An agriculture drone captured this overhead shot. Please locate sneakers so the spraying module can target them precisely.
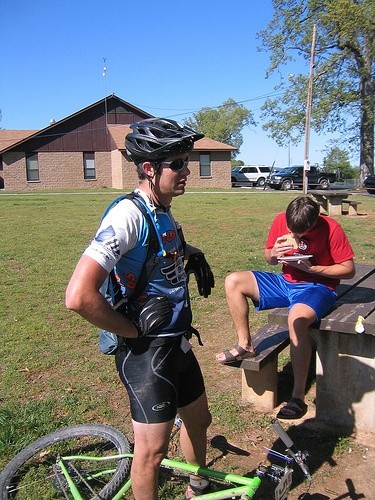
[185,481,238,500]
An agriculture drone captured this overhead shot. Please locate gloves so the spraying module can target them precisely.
[128,294,176,338]
[184,253,215,298]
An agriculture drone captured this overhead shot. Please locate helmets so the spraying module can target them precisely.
[123,117,205,162]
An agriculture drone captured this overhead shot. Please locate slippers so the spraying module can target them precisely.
[276,398,308,420]
[216,346,256,363]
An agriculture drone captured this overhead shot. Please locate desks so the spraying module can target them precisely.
[307,190,348,215]
[343,200,362,216]
[267,263,375,433]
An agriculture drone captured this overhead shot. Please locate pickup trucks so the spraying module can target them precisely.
[267,166,336,190]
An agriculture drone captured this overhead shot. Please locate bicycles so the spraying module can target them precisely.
[0,418,312,500]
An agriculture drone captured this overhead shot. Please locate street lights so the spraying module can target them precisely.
[102,57,107,134]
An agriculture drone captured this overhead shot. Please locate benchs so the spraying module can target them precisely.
[224,323,289,409]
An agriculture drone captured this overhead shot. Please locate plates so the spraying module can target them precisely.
[277,255,314,261]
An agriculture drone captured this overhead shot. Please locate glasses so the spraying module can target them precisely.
[154,156,190,171]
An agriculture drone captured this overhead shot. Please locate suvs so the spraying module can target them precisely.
[231,165,274,187]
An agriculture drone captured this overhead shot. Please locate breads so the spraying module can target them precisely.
[281,234,301,249]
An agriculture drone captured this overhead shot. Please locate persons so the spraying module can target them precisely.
[66,117,235,500]
[216,197,356,419]
